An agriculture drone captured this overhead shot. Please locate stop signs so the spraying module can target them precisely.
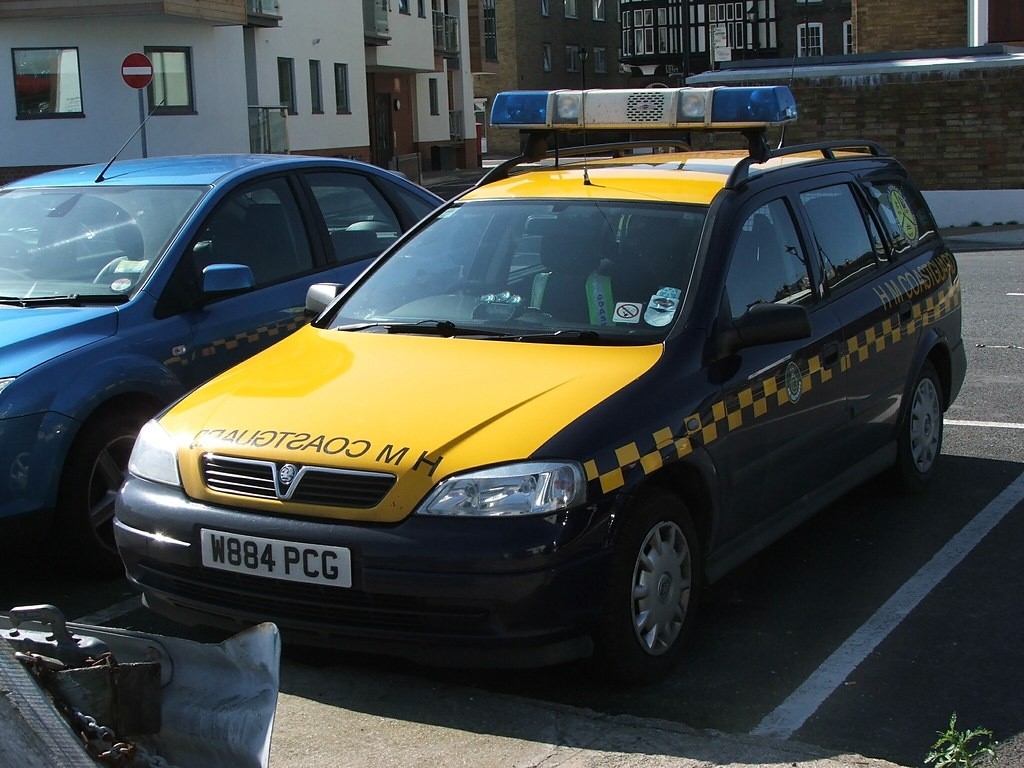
[122,52,153,90]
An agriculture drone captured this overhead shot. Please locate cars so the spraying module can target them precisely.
[116,90,966,684]
[0,152,449,563]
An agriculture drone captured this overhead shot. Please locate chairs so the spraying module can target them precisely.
[531,210,782,335]
